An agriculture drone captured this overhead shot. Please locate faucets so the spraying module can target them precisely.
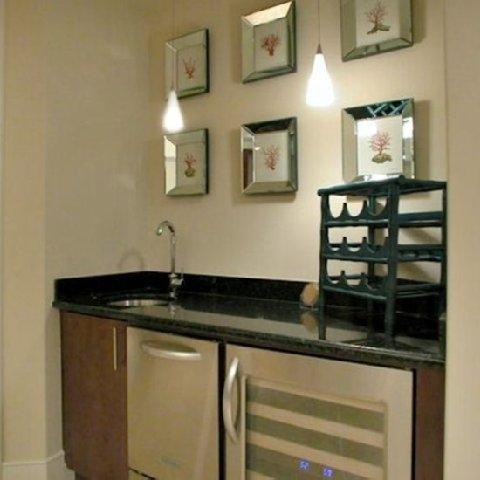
[155,221,185,292]
[166,302,179,322]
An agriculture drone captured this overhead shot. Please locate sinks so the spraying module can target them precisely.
[103,293,181,308]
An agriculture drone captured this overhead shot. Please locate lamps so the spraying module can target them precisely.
[301,0,337,109]
[162,1,186,134]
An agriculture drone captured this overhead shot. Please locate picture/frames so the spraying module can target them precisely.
[341,97,418,187]
[164,28,211,102]
[236,1,297,87]
[238,116,300,195]
[337,0,416,64]
[159,125,211,199]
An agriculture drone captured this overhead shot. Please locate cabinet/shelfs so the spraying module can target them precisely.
[57,308,128,480]
[313,178,449,348]
[219,339,415,480]
[130,323,228,479]
